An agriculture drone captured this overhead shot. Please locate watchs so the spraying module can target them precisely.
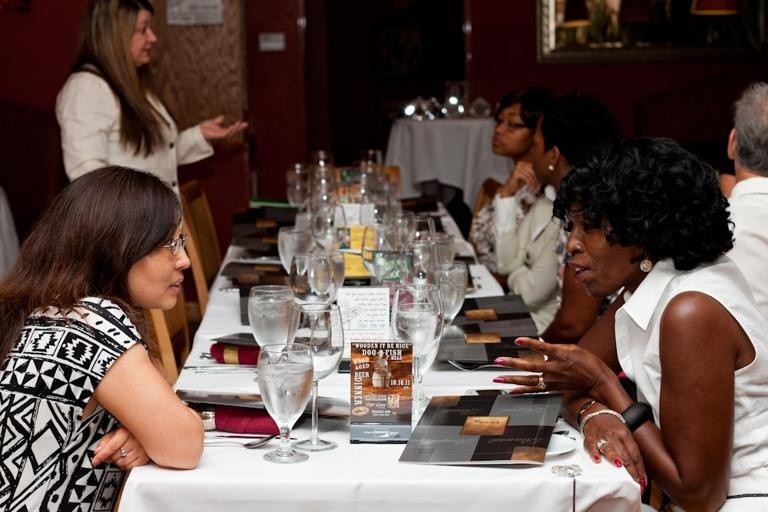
[577,399,596,426]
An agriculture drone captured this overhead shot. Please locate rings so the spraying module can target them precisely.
[535,376,548,390]
[596,438,609,450]
[120,447,127,457]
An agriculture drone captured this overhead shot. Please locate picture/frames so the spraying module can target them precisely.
[536,0,768,65]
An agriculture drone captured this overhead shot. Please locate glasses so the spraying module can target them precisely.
[494,116,526,133]
[158,233,189,256]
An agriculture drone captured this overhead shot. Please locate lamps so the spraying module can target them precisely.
[690,0,739,16]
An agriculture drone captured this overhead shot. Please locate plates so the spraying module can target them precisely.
[209,332,303,349]
[222,261,281,275]
[543,432,578,459]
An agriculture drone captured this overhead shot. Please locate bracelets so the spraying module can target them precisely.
[580,409,626,439]
[621,401,652,431]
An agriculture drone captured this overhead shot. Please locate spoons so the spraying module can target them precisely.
[201,434,278,450]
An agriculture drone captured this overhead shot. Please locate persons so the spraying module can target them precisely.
[467,83,558,266]
[1,164,202,512]
[530,92,618,343]
[724,86,768,305]
[492,137,768,511]
[492,158,557,335]
[55,0,248,207]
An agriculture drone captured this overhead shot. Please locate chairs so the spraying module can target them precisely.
[181,178,221,316]
[145,293,190,386]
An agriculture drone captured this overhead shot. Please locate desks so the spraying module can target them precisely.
[386,115,517,209]
[119,198,641,512]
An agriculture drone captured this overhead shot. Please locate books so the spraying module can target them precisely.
[400,388,566,469]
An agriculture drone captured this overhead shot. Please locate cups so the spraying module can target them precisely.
[391,283,443,421]
[383,212,415,254]
[436,260,467,327]
[256,342,314,465]
[359,226,397,288]
[358,196,390,225]
[288,302,344,453]
[432,234,455,269]
[313,251,346,297]
[290,254,335,357]
[278,226,312,277]
[310,204,346,254]
[405,217,434,245]
[247,285,295,368]
[394,302,437,408]
[403,239,433,285]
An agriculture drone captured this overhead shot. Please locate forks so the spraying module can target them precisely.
[447,358,516,372]
